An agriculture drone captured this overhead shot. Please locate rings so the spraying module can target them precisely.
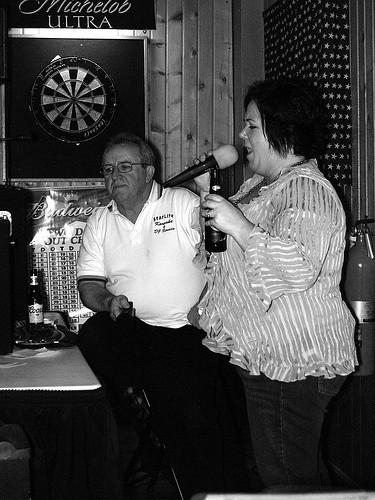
[205,211,212,218]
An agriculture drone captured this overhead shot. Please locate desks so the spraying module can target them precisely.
[0,312,107,500]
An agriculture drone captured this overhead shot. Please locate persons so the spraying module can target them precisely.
[183,77,361,497]
[77,134,250,500]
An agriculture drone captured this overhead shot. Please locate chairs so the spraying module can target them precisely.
[107,363,193,496]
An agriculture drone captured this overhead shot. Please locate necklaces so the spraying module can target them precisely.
[231,158,309,206]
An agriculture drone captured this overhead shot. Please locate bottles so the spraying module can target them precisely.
[26,269,44,343]
[205,169,226,252]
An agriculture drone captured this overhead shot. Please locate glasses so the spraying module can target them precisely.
[100,162,150,176]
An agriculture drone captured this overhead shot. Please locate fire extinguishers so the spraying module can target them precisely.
[342,216,375,377]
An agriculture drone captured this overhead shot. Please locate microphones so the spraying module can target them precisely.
[163,145,239,189]
[115,302,134,330]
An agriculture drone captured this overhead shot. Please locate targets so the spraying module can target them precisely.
[30,57,116,145]
